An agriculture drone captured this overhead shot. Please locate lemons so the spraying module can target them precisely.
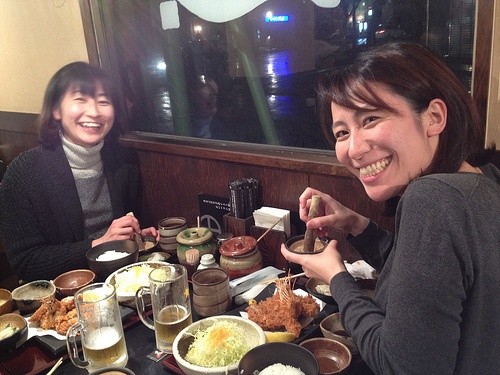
[264,331,296,343]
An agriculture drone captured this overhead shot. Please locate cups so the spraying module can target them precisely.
[191,269,233,317]
[66,282,128,374]
[135,264,193,355]
[158,216,188,261]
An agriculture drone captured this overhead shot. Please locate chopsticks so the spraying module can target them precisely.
[45,357,64,375]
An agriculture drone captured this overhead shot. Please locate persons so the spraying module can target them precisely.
[280,35,500,374]
[182,60,239,142]
[0,62,160,286]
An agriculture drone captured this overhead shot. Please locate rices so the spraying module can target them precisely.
[95,250,130,261]
[253,362,306,375]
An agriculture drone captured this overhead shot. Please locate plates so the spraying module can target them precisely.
[23,306,135,354]
[226,282,337,346]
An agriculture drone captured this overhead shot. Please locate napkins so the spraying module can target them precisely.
[344,259,378,280]
[252,206,291,238]
[229,266,287,307]
[13,295,77,349]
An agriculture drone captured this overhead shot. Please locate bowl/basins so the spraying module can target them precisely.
[54,269,95,298]
[319,312,360,355]
[173,315,267,375]
[12,280,57,314]
[86,240,138,281]
[0,314,27,349]
[238,341,319,375]
[105,261,169,309]
[0,288,13,316]
[88,367,135,375]
[306,277,336,306]
[138,252,170,265]
[299,338,352,375]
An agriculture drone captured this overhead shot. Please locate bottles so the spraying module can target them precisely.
[176,227,262,290]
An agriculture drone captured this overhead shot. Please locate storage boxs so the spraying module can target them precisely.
[222,212,255,238]
[357,278,377,291]
[251,224,304,271]
